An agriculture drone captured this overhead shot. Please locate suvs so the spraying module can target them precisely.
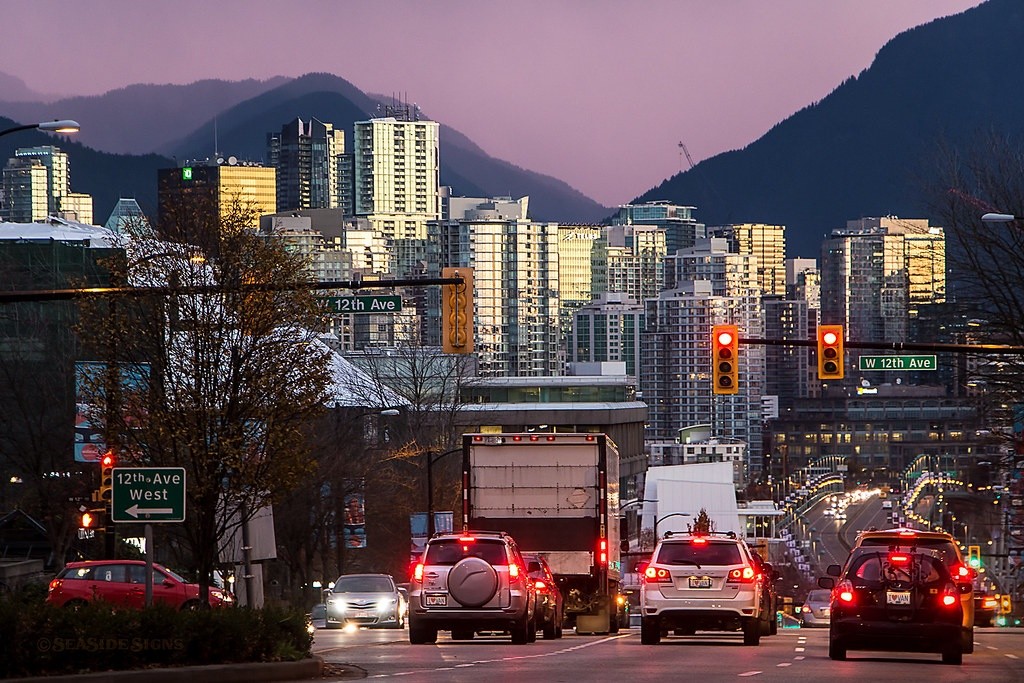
[639,531,768,646]
[409,530,541,646]
[826,526,979,654]
[43,560,236,613]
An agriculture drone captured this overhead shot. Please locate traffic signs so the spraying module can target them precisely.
[110,467,186,523]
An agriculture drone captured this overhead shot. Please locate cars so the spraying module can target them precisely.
[818,545,972,664]
[823,488,901,520]
[323,574,407,629]
[798,588,832,629]
[674,549,782,637]
[973,590,994,628]
[523,555,568,639]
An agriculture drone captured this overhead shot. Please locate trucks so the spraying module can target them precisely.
[460,432,630,636]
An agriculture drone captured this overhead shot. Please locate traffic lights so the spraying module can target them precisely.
[818,325,844,380]
[102,455,114,501]
[713,324,739,395]
[968,546,981,569]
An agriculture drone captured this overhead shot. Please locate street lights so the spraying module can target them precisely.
[653,512,691,551]
[334,400,401,577]
[770,453,847,567]
[898,453,992,551]
